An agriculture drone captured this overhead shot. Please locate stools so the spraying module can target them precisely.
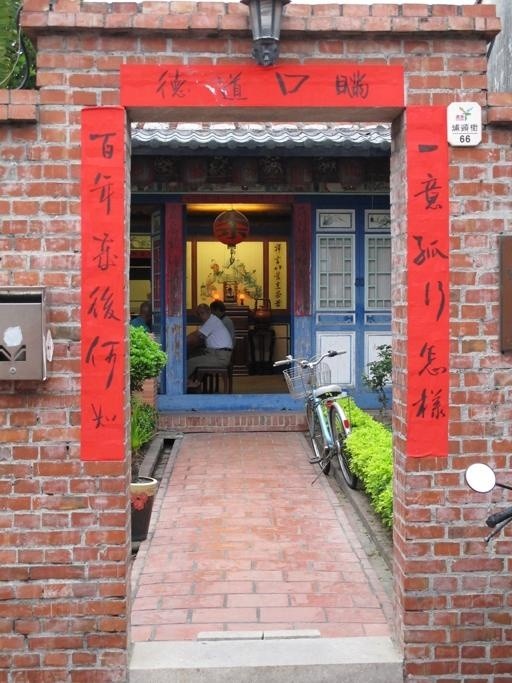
[197,367,233,393]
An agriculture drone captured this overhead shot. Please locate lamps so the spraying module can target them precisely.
[240,1,293,68]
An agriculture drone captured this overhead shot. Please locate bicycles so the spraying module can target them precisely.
[273,348,358,490]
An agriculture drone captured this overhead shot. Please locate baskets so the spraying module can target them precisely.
[283,363,331,399]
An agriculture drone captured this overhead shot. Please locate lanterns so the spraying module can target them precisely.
[213,209,249,255]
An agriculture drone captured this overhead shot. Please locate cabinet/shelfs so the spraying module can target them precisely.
[221,304,249,376]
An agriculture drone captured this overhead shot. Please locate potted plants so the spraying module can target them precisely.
[128,404,160,552]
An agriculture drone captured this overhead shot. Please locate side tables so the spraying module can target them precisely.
[246,328,275,375]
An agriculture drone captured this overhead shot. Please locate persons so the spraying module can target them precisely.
[131,300,152,332]
[186,302,233,387]
[209,298,236,347]
